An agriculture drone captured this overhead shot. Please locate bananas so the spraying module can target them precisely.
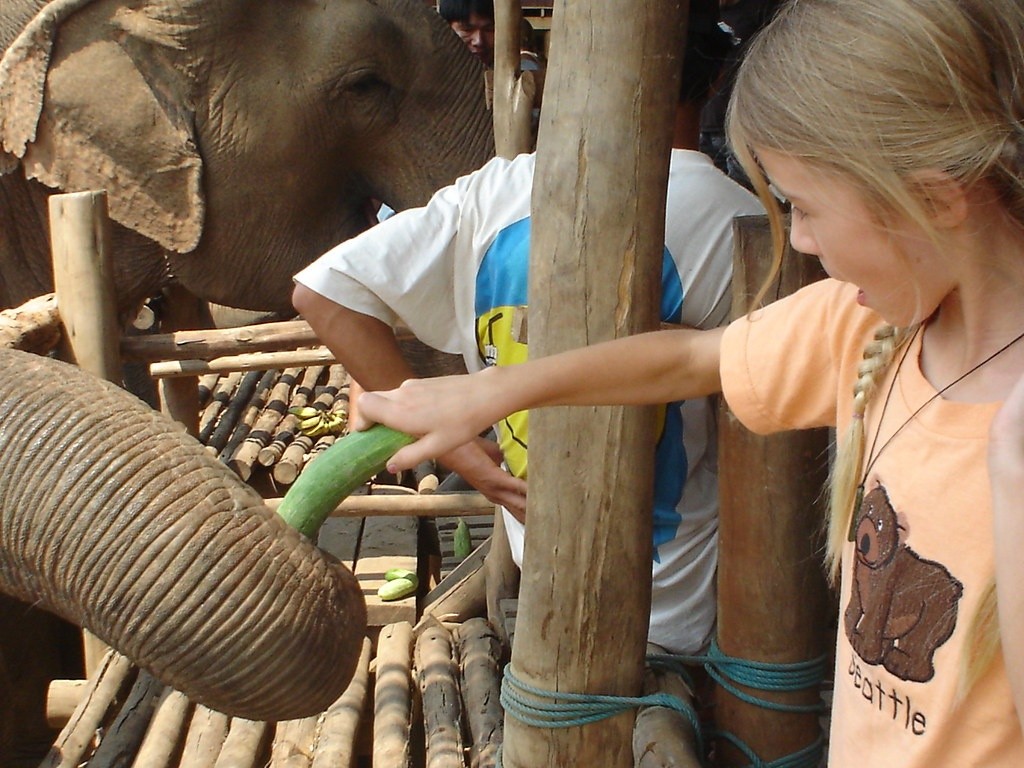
[286,406,348,435]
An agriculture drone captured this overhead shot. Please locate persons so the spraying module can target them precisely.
[348,0,1023,768]
[442,0,783,197]
[293,8,774,665]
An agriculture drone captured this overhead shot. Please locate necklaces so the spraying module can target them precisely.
[844,312,1022,540]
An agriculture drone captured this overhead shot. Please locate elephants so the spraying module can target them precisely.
[0,345,370,768]
[0,1,495,358]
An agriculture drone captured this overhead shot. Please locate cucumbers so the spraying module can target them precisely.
[277,424,419,541]
[454,520,471,558]
[378,569,419,600]
